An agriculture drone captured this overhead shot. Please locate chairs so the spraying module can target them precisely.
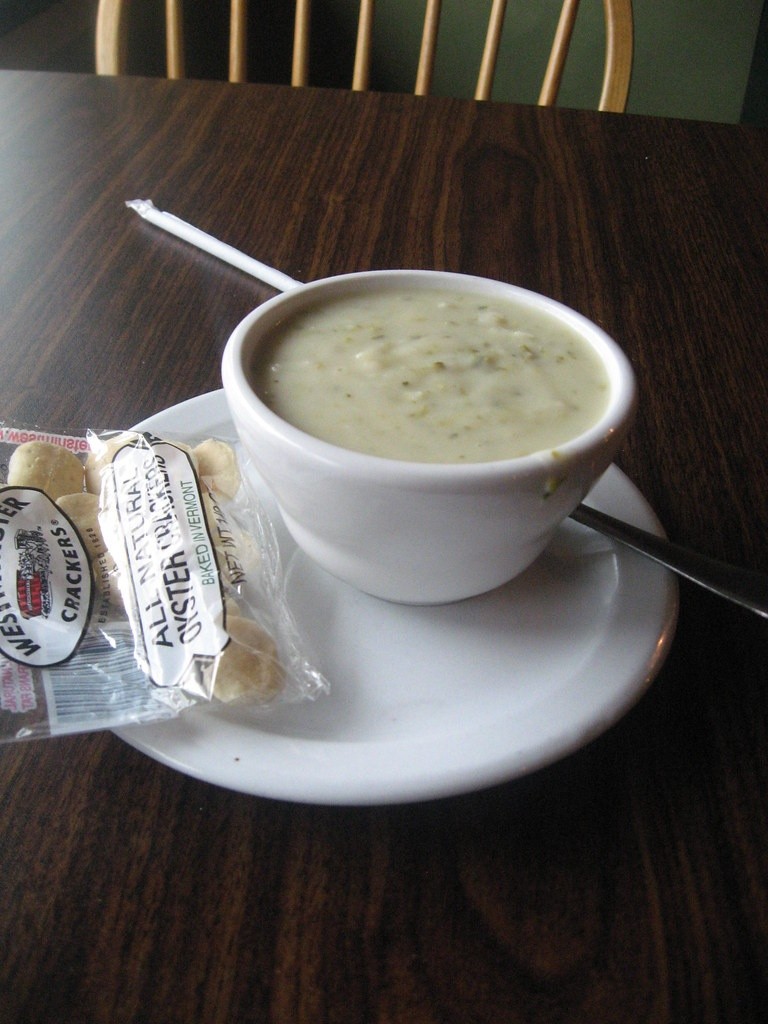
[94,1,636,113]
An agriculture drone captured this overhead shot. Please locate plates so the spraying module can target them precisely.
[109,384,684,805]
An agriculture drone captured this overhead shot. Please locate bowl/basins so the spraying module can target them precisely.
[219,270,640,606]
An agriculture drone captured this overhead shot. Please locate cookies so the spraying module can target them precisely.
[6,435,285,707]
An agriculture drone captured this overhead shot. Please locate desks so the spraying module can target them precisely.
[0,67,768,1024]
